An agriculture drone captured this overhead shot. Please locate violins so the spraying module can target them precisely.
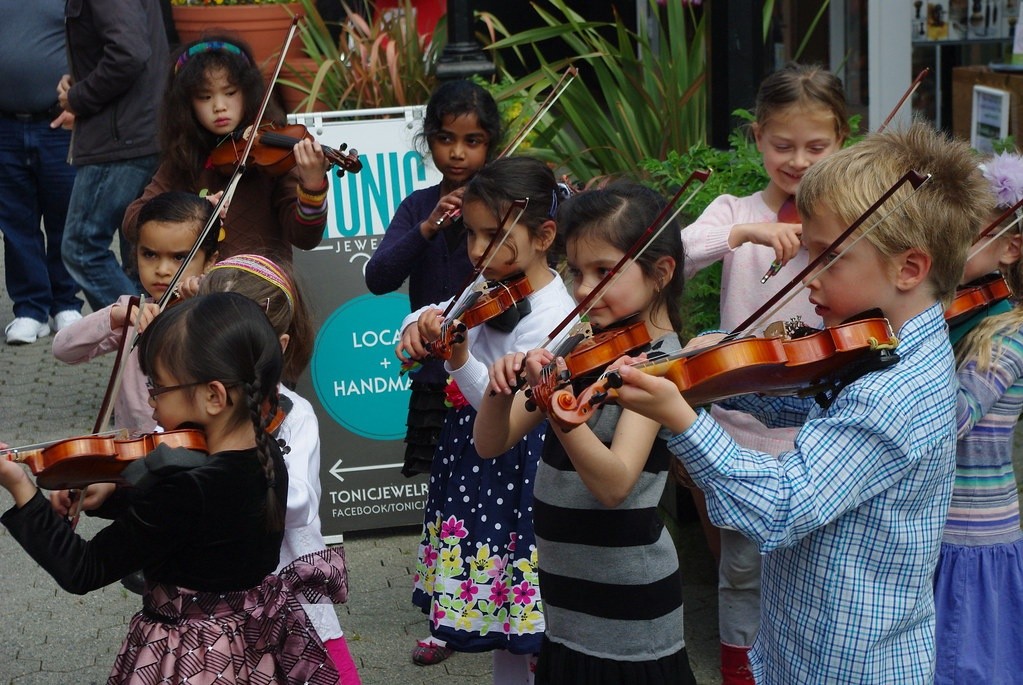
[944,269,1013,327]
[778,194,803,247]
[524,319,653,413]
[0,427,210,490]
[545,316,902,435]
[211,118,363,179]
[425,275,535,361]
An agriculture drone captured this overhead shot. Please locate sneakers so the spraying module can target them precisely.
[51,310,83,334]
[4,315,50,344]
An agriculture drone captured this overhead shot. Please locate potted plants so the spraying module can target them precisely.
[641,101,868,562]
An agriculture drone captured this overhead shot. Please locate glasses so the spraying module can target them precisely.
[144,381,234,407]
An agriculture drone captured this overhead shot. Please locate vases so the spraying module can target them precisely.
[172,2,333,112]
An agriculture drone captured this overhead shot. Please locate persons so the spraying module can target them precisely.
[51,0,177,310]
[677,64,848,685]
[473,178,697,685]
[364,79,501,665]
[605,118,992,685]
[51,191,223,438]
[0,294,349,685]
[121,31,331,264]
[931,154,1023,685]
[397,154,586,685]
[0,0,83,344]
[196,251,363,685]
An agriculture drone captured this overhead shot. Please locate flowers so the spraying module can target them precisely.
[170,0,297,6]
[978,147,1023,208]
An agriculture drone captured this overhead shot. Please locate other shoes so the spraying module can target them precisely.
[413,633,454,666]
